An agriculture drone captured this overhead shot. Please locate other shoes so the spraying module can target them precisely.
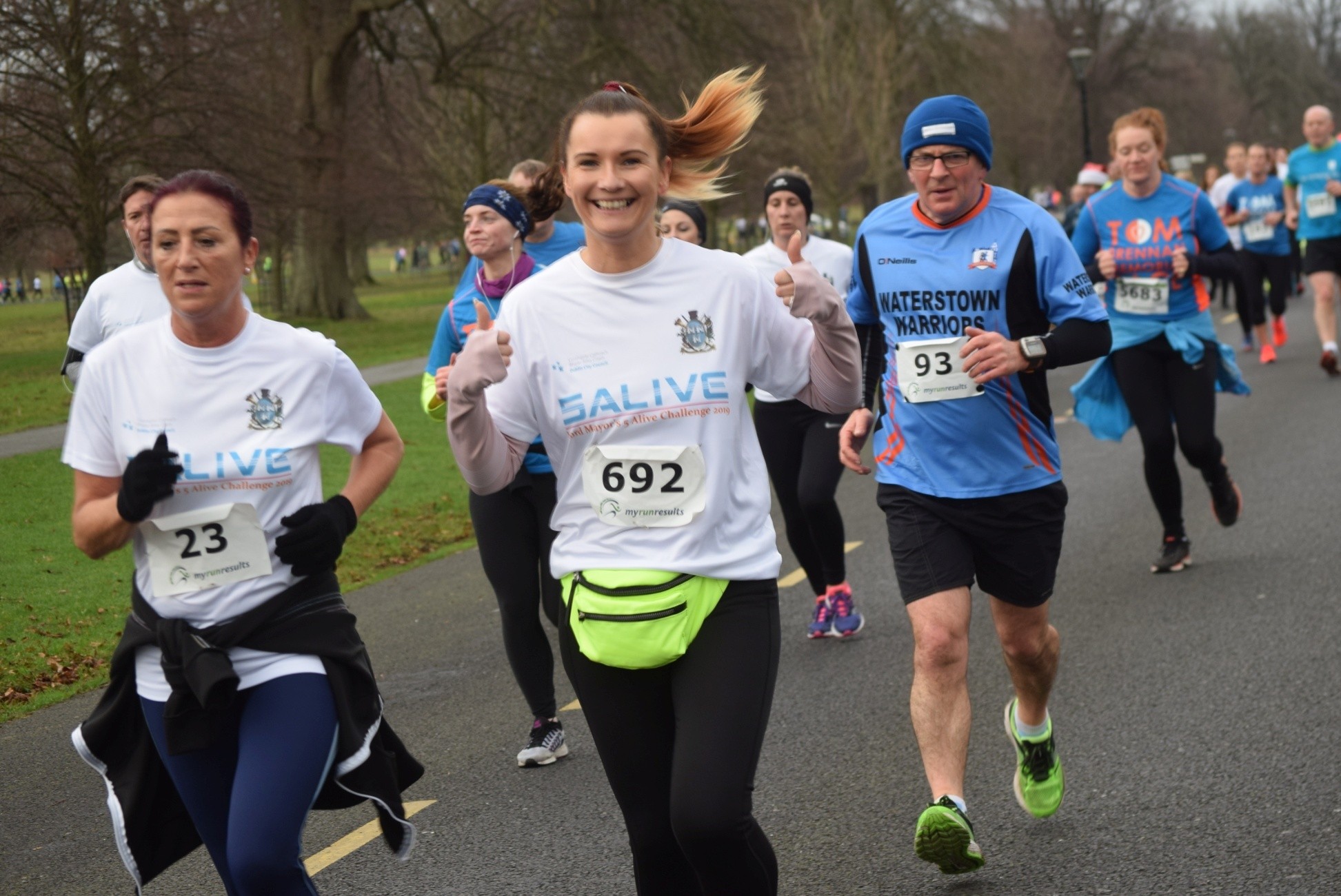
[1321,350,1341,375]
[1271,316,1285,347]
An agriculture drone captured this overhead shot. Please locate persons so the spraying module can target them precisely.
[0,277,14,302]
[55,273,65,296]
[61,172,406,896]
[32,276,43,299]
[735,166,866,641]
[1027,163,1112,238]
[1072,108,1242,575]
[1164,141,1308,365]
[1283,104,1341,378]
[60,176,253,380]
[394,237,460,269]
[419,167,570,767]
[453,159,585,297]
[839,95,1113,874]
[447,68,863,896]
[15,277,24,301]
[659,198,706,247]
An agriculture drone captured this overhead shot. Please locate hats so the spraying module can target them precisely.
[900,95,991,171]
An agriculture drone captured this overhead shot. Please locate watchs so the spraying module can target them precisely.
[1020,336,1047,372]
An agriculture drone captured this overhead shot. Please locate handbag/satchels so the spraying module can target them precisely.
[1259,344,1276,364]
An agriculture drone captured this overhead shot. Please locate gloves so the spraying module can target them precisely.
[116,433,183,524]
[272,493,361,577]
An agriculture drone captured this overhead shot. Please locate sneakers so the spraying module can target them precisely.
[913,797,985,874]
[1203,466,1242,527]
[515,714,570,766]
[1002,700,1064,818]
[1149,535,1190,574]
[806,595,833,638]
[824,583,863,638]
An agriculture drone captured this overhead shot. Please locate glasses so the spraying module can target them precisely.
[907,150,972,171]
